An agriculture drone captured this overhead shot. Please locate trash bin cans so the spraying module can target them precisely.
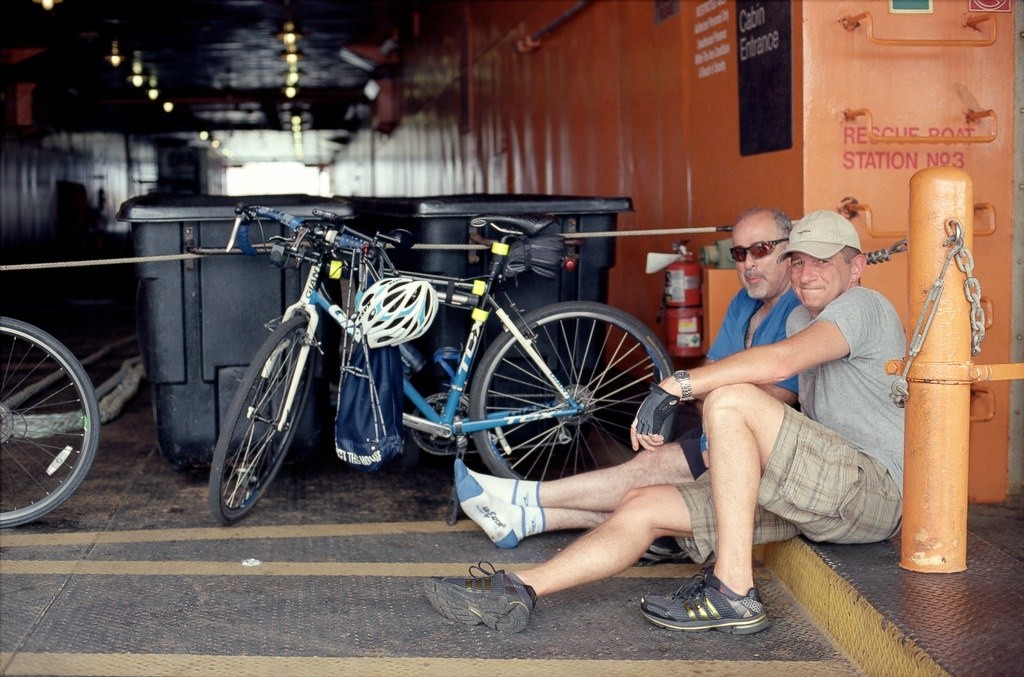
[351,195,636,483]
[115,192,359,472]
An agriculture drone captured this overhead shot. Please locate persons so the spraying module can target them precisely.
[454,208,813,563]
[423,209,909,635]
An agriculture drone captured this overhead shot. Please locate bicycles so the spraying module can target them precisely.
[208,200,680,525]
[0,317,102,528]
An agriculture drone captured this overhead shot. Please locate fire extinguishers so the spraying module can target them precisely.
[657,240,704,358]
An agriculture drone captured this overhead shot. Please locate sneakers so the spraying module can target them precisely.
[639,566,771,633]
[425,560,539,632]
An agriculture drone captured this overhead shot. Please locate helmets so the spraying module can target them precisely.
[357,278,439,348]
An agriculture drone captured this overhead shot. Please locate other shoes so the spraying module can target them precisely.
[642,534,694,565]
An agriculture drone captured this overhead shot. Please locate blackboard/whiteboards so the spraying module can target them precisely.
[736,0,793,157]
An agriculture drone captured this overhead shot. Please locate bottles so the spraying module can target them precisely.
[370,315,427,378]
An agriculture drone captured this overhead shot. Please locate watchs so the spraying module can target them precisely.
[673,369,692,401]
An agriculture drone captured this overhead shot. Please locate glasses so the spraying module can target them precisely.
[730,238,790,262]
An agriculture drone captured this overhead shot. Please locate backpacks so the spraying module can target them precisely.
[334,249,405,467]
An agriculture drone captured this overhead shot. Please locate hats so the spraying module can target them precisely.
[777,210,862,265]
